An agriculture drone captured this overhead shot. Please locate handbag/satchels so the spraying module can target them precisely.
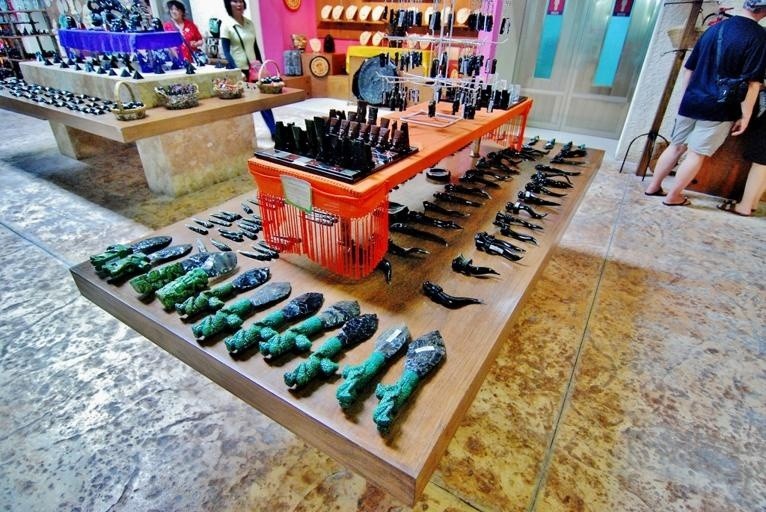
[191,49,208,64]
[717,77,747,102]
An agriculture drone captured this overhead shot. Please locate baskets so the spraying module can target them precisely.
[667,27,704,48]
[110,60,285,120]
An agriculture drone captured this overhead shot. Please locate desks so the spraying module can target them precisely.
[0,80,306,197]
[66,139,607,510]
[58,28,183,72]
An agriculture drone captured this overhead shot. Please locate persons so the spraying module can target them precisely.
[645,0,766,216]
[220,0,279,141]
[163,0,204,62]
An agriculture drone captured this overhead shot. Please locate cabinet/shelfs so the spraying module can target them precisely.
[0,9,62,78]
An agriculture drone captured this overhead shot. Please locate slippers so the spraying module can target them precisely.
[645,187,691,206]
[717,200,756,216]
